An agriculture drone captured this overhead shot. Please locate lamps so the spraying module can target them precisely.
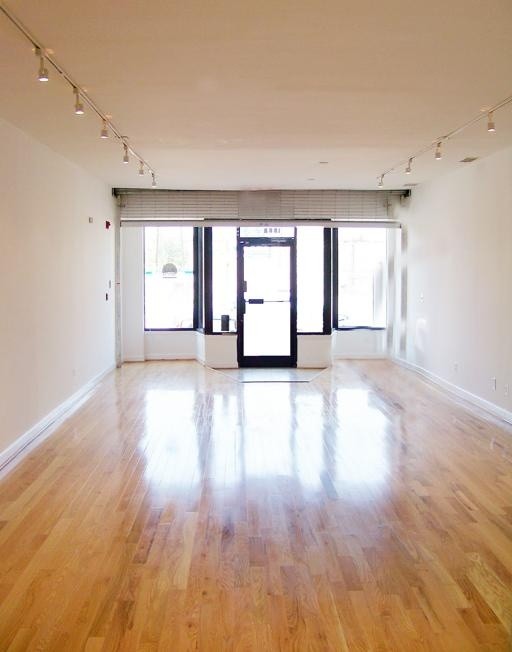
[0,3,161,188]
[372,97,512,188]
